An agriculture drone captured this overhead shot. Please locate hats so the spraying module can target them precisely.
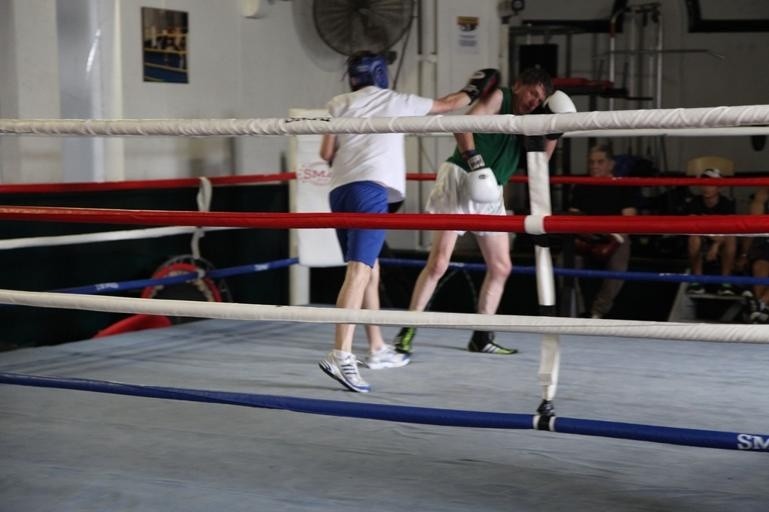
[701,167,722,178]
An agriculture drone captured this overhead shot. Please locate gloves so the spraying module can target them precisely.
[540,89,579,140]
[460,68,502,106]
[461,149,502,204]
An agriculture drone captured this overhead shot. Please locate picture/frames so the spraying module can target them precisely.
[684,1,769,34]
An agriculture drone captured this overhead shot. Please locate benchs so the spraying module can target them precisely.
[456,243,688,264]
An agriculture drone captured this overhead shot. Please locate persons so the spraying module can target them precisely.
[568,141,639,319]
[394,64,578,355]
[686,168,738,296]
[736,186,769,323]
[318,51,502,394]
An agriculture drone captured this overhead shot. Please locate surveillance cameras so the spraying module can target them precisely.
[498,0,526,18]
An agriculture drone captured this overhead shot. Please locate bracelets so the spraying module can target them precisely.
[741,253,747,257]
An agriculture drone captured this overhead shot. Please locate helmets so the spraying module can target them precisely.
[347,51,389,92]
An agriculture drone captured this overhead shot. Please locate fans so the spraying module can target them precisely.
[313,0,414,57]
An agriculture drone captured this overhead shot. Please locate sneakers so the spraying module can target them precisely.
[468,336,518,355]
[362,342,413,370]
[318,348,372,394]
[687,281,769,324]
[392,327,417,352]
